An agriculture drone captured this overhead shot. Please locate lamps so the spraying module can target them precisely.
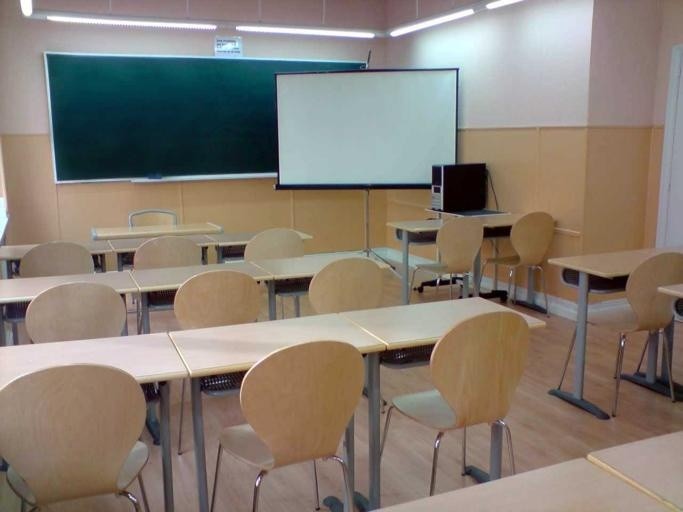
[16,1,520,40]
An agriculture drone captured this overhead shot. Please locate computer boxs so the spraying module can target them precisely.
[432,163,486,210]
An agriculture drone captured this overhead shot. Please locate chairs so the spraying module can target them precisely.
[2,212,681,512]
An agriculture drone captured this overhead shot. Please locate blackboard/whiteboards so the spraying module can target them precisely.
[43,51,369,184]
